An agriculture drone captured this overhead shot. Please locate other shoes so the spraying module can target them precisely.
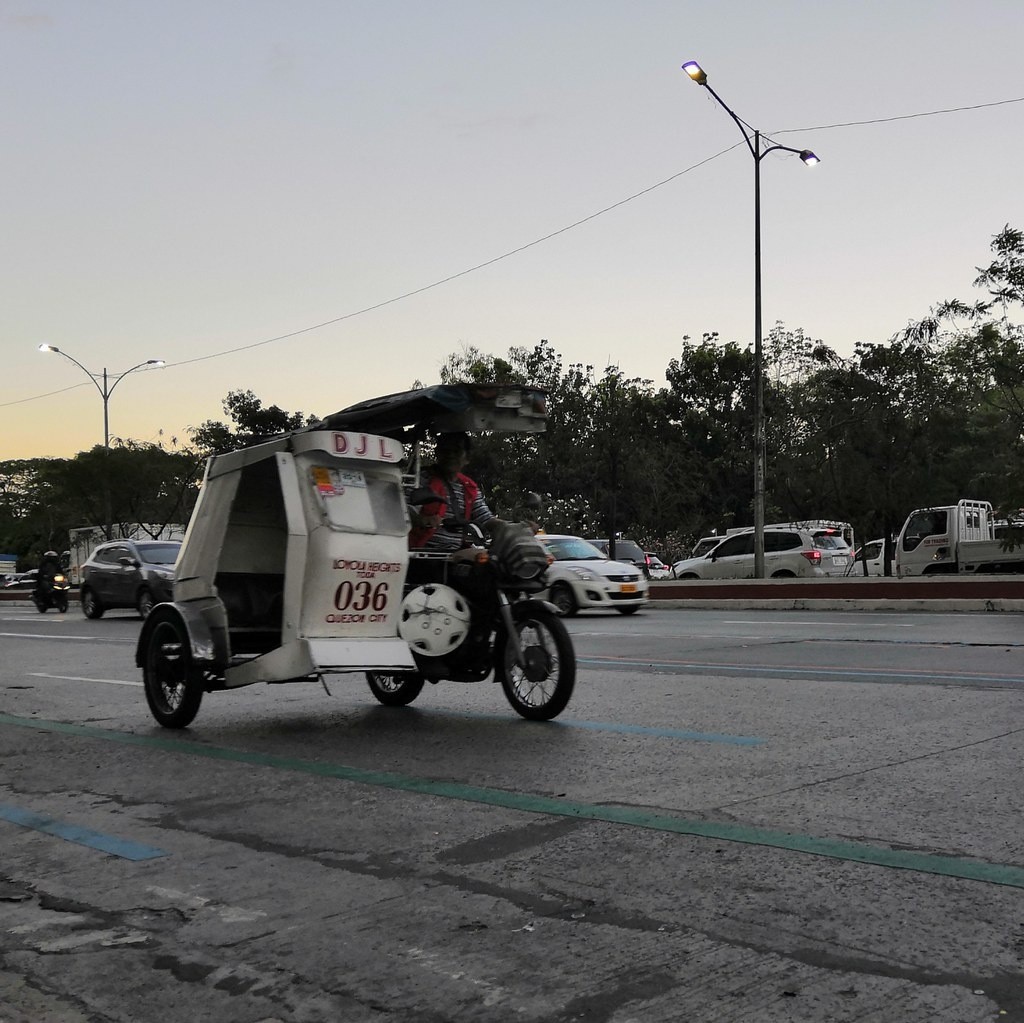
[413,653,450,677]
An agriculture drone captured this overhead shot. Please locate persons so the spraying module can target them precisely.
[406,432,513,677]
[35,551,65,606]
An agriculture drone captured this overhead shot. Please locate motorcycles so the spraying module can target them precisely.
[136,431,576,729]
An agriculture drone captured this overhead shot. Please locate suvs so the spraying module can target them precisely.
[668,526,824,580]
[79,538,186,619]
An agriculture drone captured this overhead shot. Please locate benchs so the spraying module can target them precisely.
[214,570,286,646]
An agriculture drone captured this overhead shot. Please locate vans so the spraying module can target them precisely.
[855,536,920,576]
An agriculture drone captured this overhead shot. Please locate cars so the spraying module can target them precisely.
[534,535,667,618]
[3,569,70,589]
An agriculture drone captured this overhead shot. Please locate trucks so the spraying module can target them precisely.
[895,499,1024,576]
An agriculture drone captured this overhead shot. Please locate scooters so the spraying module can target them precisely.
[26,569,72,613]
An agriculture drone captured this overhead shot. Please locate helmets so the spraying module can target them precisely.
[44,551,58,557]
